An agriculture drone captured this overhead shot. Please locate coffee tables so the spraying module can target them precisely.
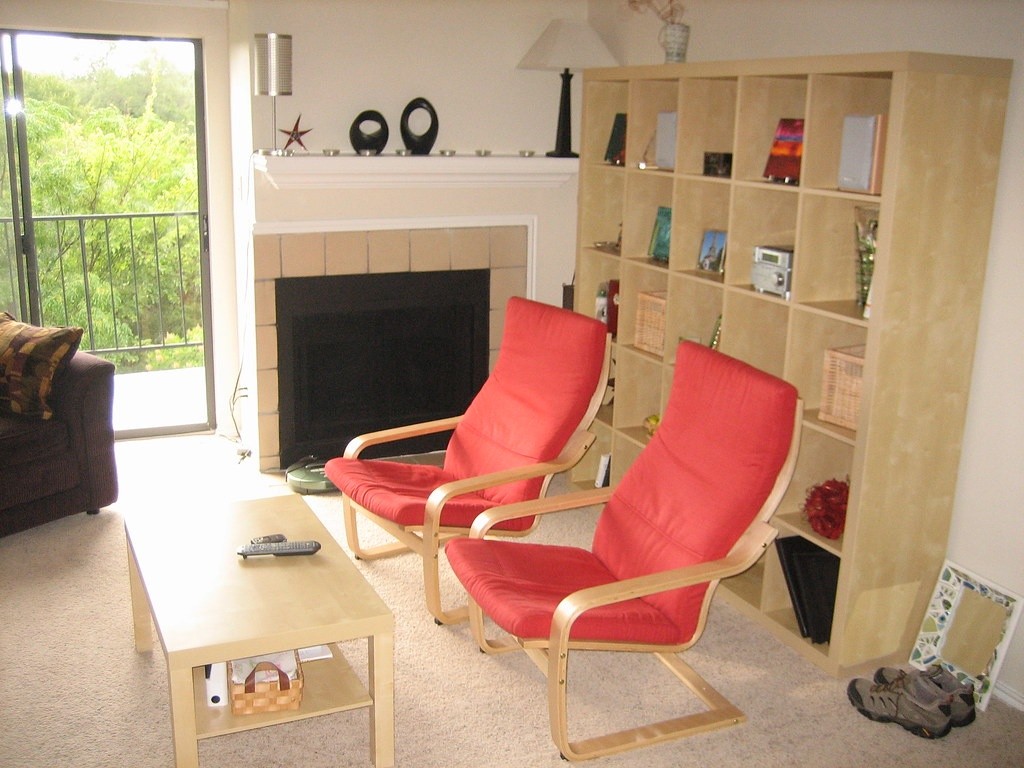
[124,493,394,768]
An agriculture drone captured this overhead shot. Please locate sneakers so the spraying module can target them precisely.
[847,678,952,739]
[874,664,976,727]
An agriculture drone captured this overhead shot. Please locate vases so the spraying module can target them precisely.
[658,22,691,63]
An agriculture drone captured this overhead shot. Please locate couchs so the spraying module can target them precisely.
[0,351,119,538]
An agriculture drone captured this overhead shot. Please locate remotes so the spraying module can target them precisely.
[236,541,321,559]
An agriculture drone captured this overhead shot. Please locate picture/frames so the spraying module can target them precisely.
[695,227,727,276]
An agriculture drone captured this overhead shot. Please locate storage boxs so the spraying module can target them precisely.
[818,344,866,432]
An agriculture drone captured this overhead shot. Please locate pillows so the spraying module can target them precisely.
[0,311,84,420]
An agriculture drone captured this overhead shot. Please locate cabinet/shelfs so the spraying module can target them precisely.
[572,50,1014,684]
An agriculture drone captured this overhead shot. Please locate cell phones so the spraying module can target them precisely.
[251,534,287,544]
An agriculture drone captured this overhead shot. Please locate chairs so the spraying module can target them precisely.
[444,339,805,762]
[323,296,614,626]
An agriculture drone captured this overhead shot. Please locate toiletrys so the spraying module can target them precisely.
[594,279,620,338]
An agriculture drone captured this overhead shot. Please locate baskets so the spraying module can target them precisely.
[226,649,304,716]
[818,344,866,430]
[634,291,667,357]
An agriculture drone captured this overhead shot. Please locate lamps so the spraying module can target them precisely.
[253,33,295,157]
[515,18,621,158]
